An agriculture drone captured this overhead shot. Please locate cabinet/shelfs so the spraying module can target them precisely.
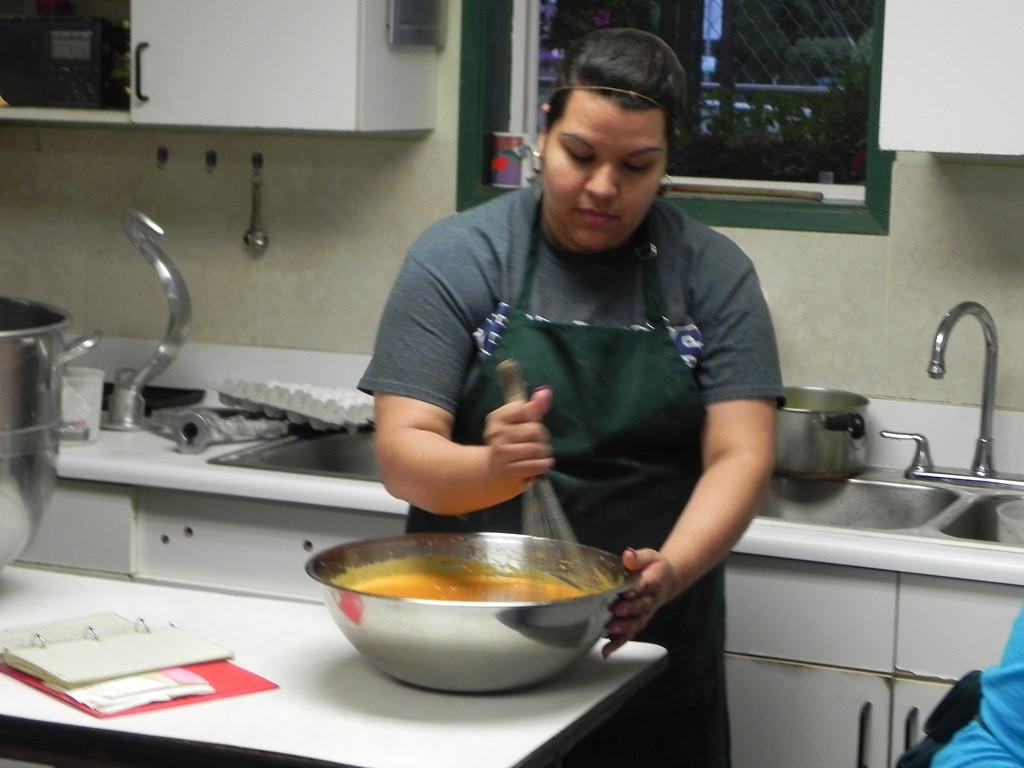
[876,0,1024,156]
[130,0,435,133]
[723,555,1024,768]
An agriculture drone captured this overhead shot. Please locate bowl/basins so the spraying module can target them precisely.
[306,532,640,692]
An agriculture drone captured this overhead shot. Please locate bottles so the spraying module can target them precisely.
[111,20,129,109]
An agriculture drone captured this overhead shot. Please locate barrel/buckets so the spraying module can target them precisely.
[996,500,1024,547]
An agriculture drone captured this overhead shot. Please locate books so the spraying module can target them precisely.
[0,611,279,722]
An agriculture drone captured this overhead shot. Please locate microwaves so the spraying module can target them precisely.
[0,16,101,110]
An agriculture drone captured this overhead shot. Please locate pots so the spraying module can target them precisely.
[0,296,106,570]
[775,387,870,479]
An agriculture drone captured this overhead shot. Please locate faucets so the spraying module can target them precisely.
[879,301,1024,490]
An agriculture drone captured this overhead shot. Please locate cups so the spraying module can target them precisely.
[492,132,533,189]
[59,366,104,447]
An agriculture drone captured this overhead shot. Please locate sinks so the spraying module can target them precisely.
[205,427,383,488]
[924,480,1024,554]
[754,466,976,534]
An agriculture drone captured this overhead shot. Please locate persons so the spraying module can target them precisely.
[935,592,1024,768]
[358,26,785,768]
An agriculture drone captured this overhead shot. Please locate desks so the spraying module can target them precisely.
[0,558,673,768]
